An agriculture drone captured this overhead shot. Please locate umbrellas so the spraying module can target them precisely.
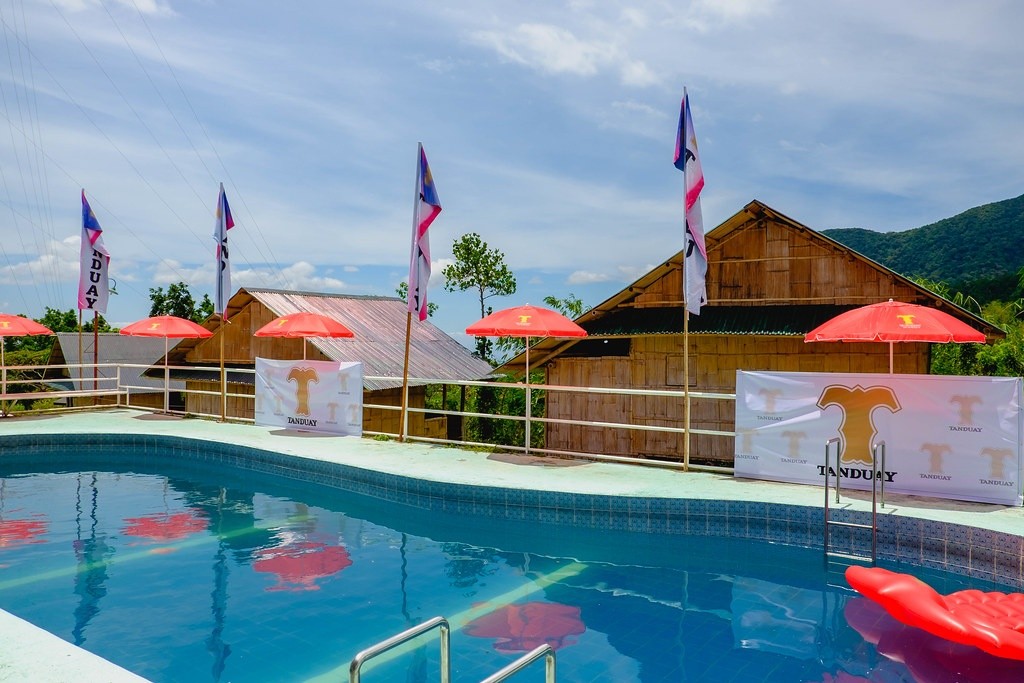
[0,313,53,366]
[253,312,354,360]
[120,316,213,413]
[802,298,986,375]
[465,303,588,455]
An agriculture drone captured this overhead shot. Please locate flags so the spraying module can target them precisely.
[78,193,110,313]
[673,95,708,314]
[406,146,441,321]
[214,189,235,320]
